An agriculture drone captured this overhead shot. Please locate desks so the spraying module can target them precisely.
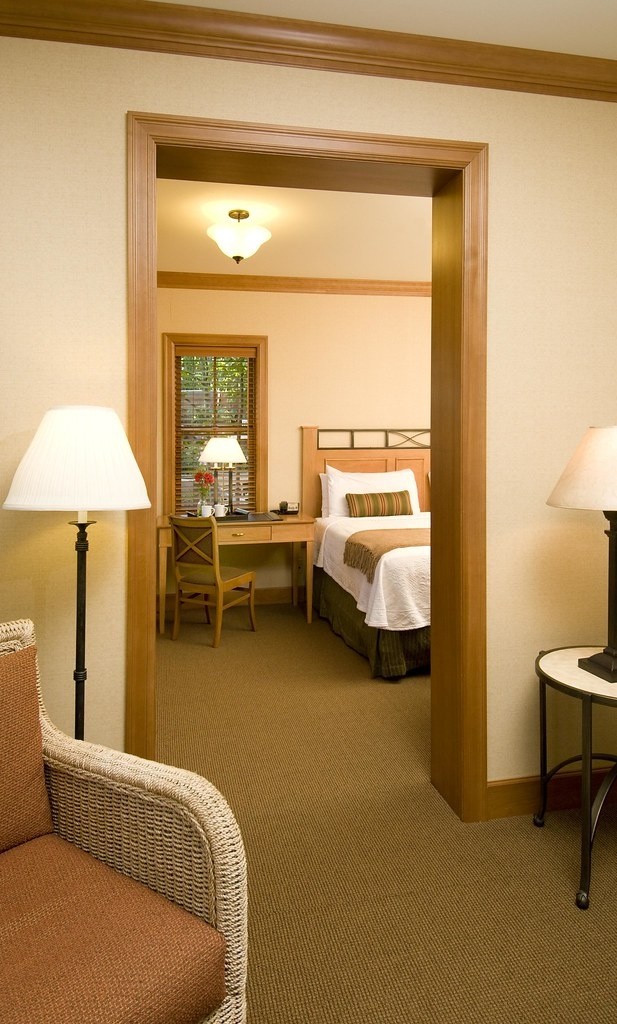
[535,646,617,910]
[157,510,317,630]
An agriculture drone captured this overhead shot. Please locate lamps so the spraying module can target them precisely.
[206,209,271,266]
[544,425,617,683]
[197,435,247,520]
[1,406,153,741]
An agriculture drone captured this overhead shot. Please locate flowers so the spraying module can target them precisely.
[192,471,214,499]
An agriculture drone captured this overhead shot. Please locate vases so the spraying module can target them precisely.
[197,493,207,516]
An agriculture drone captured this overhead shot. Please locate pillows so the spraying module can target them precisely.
[324,464,420,517]
[319,473,330,518]
[346,489,413,517]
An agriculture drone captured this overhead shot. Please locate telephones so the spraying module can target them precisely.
[279,501,299,514]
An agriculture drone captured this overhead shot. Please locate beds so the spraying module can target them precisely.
[298,426,432,679]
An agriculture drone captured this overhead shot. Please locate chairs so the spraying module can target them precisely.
[166,514,256,648]
[0,619,249,1024]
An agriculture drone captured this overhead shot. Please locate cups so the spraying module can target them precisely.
[214,504,228,517]
[202,506,216,517]
